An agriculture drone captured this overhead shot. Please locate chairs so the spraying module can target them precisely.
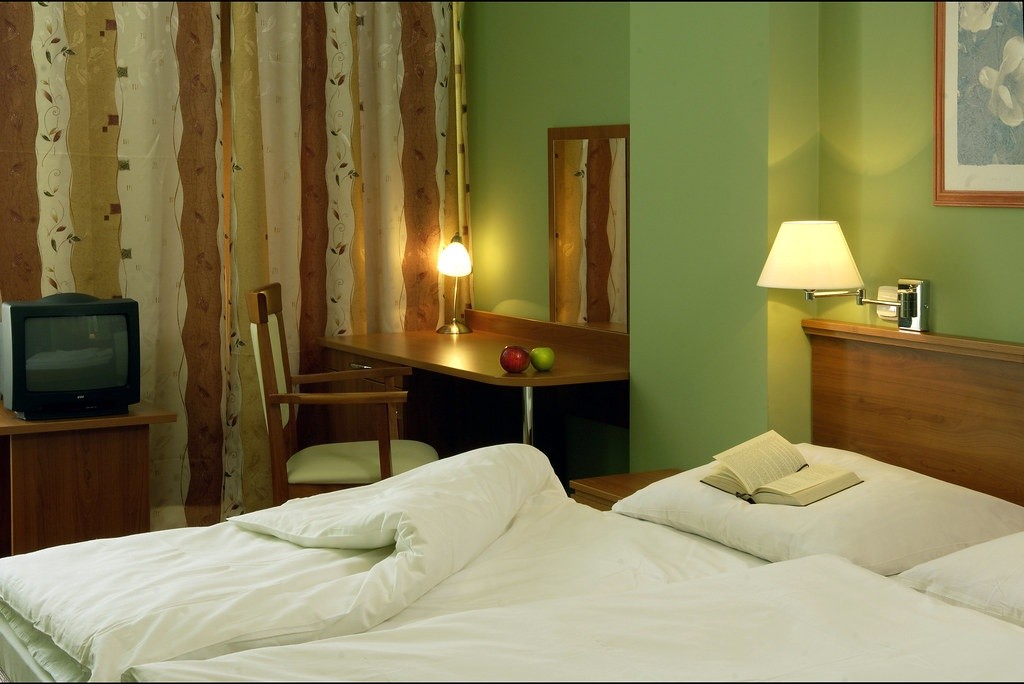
[252,282,440,507]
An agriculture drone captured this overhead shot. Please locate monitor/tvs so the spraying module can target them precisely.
[0,292,142,422]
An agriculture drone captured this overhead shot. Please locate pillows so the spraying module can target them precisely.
[612,440,1024,578]
[886,530,1024,630]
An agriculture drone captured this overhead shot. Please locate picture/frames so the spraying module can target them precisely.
[932,1,1023,210]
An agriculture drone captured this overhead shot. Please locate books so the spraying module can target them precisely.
[700,430,864,507]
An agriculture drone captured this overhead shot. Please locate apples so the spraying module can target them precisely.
[500,345,531,373]
[531,347,555,371]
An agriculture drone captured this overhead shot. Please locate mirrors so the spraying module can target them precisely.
[547,123,630,335]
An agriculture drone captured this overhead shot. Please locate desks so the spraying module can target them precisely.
[0,403,178,558]
[315,331,629,489]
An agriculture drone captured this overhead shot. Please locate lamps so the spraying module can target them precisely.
[753,220,930,337]
[438,232,474,334]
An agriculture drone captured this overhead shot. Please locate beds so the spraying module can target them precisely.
[0,320,1024,683]
